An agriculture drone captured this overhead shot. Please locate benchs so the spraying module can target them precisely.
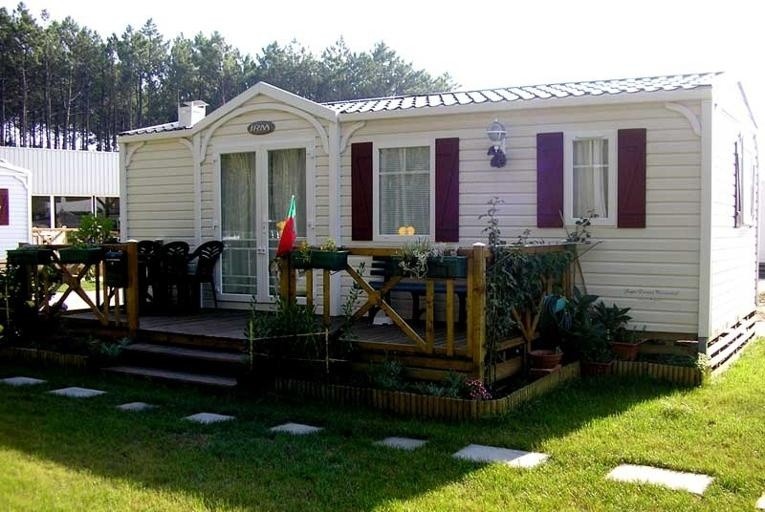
[367,253,467,325]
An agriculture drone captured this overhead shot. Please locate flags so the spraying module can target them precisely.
[275,198,297,255]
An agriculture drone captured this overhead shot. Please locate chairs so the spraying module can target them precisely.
[136,239,224,317]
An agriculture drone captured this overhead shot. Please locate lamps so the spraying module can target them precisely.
[486,118,509,156]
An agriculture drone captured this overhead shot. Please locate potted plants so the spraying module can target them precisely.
[576,302,647,376]
[292,238,349,270]
[58,213,103,264]
